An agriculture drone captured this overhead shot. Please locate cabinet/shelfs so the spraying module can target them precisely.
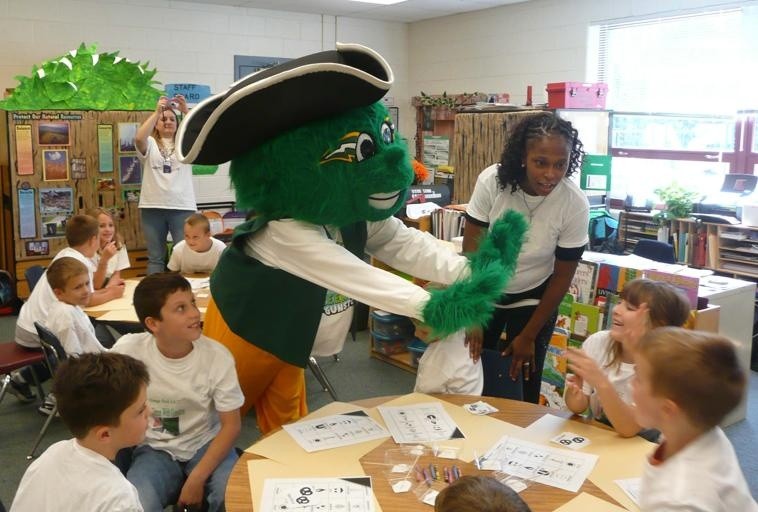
[718,221,757,372]
[369,214,433,377]
[616,211,718,272]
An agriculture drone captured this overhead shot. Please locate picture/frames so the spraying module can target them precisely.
[97,122,115,175]
[118,155,143,186]
[18,188,37,240]
[116,122,140,153]
[15,122,35,176]
[39,122,71,147]
[39,188,75,239]
[42,150,70,183]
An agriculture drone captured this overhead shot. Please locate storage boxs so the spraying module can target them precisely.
[545,81,611,111]
[406,341,429,367]
[370,311,415,339]
[370,330,415,357]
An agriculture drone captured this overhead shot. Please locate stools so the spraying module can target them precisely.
[1,343,46,407]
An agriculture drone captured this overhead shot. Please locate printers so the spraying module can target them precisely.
[691,173,758,225]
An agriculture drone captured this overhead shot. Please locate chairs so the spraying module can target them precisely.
[26,320,66,460]
[25,264,44,292]
[306,352,341,401]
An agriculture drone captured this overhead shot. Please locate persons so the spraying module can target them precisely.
[165,213,226,275]
[466,108,589,405]
[433,474,532,512]
[630,326,757,511]
[108,272,244,511]
[563,277,690,442]
[47,257,109,356]
[8,352,151,511]
[85,208,132,290]
[133,93,196,278]
[173,40,534,444]
[13,215,127,351]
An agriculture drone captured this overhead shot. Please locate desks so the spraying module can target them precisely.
[84,271,212,328]
[224,395,658,511]
[576,250,757,429]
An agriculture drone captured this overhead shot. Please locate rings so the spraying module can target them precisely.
[523,362,530,368]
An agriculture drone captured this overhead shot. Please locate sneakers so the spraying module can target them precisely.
[1,374,37,403]
[39,396,60,417]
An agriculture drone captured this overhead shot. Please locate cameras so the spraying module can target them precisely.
[164,98,180,110]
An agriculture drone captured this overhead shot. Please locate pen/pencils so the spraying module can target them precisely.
[415,463,462,488]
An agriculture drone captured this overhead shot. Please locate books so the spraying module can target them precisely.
[537,259,701,412]
[623,217,758,274]
[428,208,466,243]
